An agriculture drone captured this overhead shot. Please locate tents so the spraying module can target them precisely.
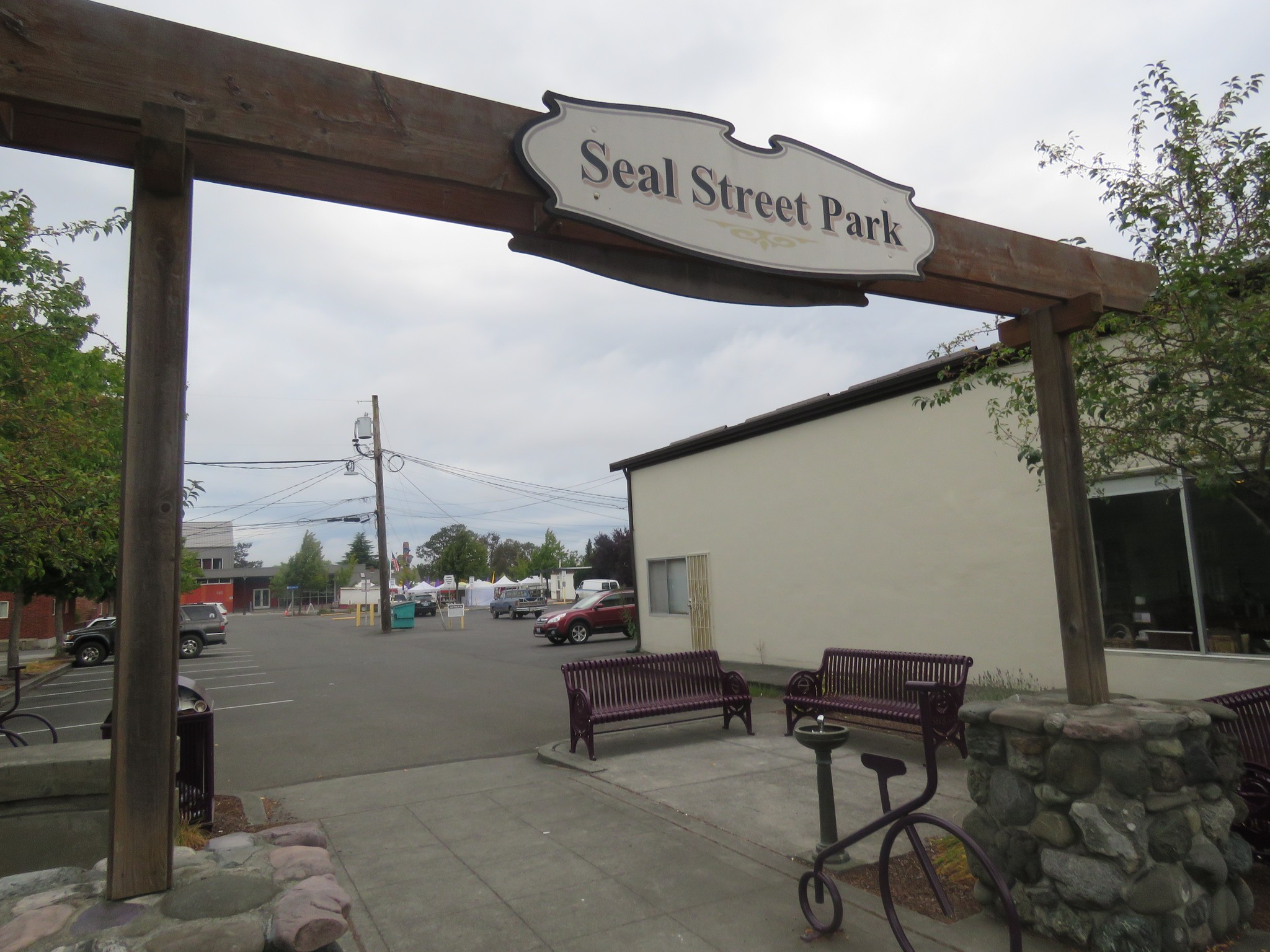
[408,581,466,597]
[518,575,551,592]
[494,575,518,594]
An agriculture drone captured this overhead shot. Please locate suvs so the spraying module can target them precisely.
[406,593,438,616]
[389,594,407,604]
[186,602,228,625]
[59,602,225,667]
[533,589,635,645]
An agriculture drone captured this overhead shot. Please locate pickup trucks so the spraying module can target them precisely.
[490,589,548,620]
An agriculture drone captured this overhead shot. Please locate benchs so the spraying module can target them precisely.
[561,650,755,762]
[783,648,973,768]
[1201,685,1270,864]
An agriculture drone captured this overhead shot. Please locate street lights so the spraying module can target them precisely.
[360,573,368,623]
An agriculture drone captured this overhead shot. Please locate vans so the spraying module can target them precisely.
[575,579,620,606]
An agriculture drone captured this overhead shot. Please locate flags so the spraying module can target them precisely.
[391,551,400,571]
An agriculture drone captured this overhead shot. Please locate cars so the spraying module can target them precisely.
[82,615,118,626]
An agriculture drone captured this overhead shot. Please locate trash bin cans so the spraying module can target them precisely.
[390,601,415,628]
[100,675,215,832]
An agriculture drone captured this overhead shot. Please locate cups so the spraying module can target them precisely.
[194,700,207,713]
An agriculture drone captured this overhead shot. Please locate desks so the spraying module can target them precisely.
[1208,634,1250,654]
